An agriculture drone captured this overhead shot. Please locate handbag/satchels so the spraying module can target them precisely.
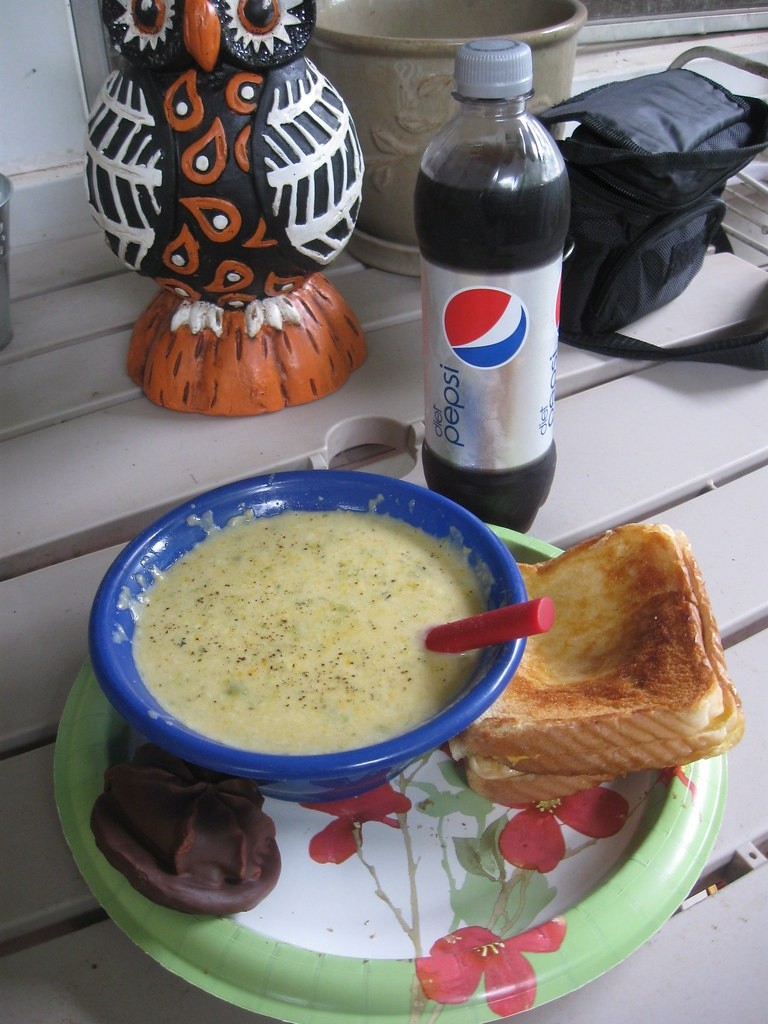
[535,69,767,342]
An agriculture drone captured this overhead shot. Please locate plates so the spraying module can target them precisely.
[53,523,730,1024]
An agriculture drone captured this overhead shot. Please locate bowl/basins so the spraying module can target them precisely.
[90,470,530,803]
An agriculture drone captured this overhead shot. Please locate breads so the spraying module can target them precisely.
[449,521,747,806]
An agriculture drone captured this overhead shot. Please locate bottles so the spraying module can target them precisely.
[414,37,570,532]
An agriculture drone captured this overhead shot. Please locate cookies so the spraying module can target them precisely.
[90,742,282,915]
